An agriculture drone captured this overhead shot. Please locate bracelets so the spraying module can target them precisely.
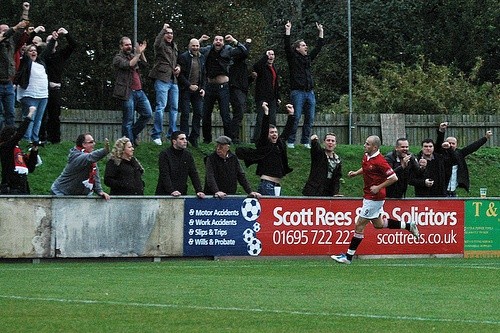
[22,13,28,16]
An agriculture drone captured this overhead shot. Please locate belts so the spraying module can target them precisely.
[260,179,281,187]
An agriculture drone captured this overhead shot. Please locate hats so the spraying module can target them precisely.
[215,136,232,144]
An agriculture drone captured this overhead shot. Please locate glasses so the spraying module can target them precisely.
[85,140,95,143]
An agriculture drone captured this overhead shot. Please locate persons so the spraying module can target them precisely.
[331,135,419,264]
[383,138,427,197]
[104,137,145,195]
[284,20,324,149]
[180,38,207,150]
[302,133,343,197]
[415,138,458,198]
[0,106,39,195]
[50,132,110,201]
[148,23,181,146]
[199,34,257,144]
[155,131,205,199]
[255,102,294,196]
[435,122,493,197]
[113,36,152,148]
[203,136,262,198]
[251,49,282,147]
[0,1,78,151]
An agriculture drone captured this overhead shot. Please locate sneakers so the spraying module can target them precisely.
[409,221,419,238]
[330,254,352,265]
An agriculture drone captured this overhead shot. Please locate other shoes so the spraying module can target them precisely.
[300,143,311,149]
[286,140,295,148]
[153,138,162,146]
